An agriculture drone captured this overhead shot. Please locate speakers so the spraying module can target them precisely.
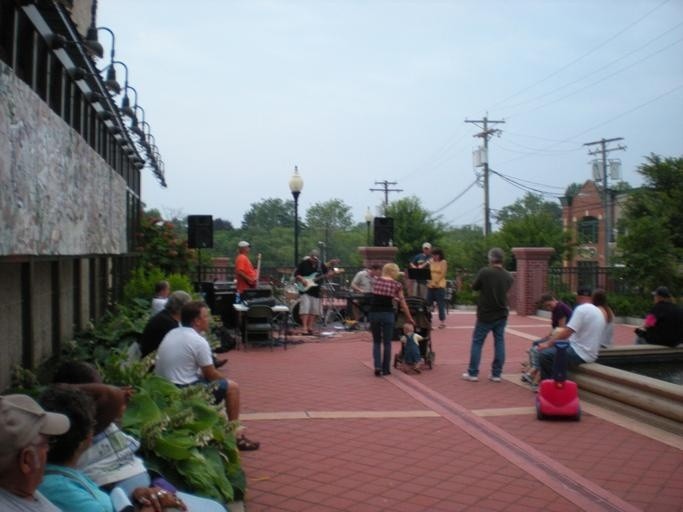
[372,216,395,247]
[186,214,214,250]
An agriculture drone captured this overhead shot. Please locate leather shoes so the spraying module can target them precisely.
[383,371,391,375]
[375,368,381,376]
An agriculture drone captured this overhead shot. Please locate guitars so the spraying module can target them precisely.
[294,268,344,293]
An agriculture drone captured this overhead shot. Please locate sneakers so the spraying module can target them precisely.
[489,376,502,382]
[531,382,538,393]
[462,373,479,382]
[521,373,533,384]
[235,434,259,451]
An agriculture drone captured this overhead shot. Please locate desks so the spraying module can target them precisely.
[334,289,367,318]
[233,303,290,349]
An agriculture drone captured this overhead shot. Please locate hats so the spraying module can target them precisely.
[238,241,250,247]
[577,287,592,296]
[489,248,503,257]
[310,249,320,258]
[646,315,657,327]
[174,290,192,306]
[423,242,431,249]
[1,393,72,457]
[651,286,671,296]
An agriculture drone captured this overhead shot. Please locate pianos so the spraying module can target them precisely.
[336,289,372,300]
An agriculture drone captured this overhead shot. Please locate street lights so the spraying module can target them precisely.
[362,205,375,246]
[287,165,309,266]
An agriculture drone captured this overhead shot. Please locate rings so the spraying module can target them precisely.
[138,488,167,503]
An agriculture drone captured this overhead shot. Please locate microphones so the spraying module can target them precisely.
[313,255,320,263]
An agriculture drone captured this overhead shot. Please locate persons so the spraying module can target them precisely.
[635,286,683,345]
[35,385,187,512]
[151,280,227,369]
[519,285,614,393]
[0,394,63,512]
[351,241,447,376]
[294,250,334,334]
[156,302,260,451]
[52,361,227,512]
[140,291,192,370]
[234,241,259,336]
[462,248,513,382]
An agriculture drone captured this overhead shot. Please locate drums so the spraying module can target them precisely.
[291,299,304,325]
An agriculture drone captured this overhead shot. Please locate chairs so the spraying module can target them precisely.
[244,305,273,352]
[278,300,303,343]
